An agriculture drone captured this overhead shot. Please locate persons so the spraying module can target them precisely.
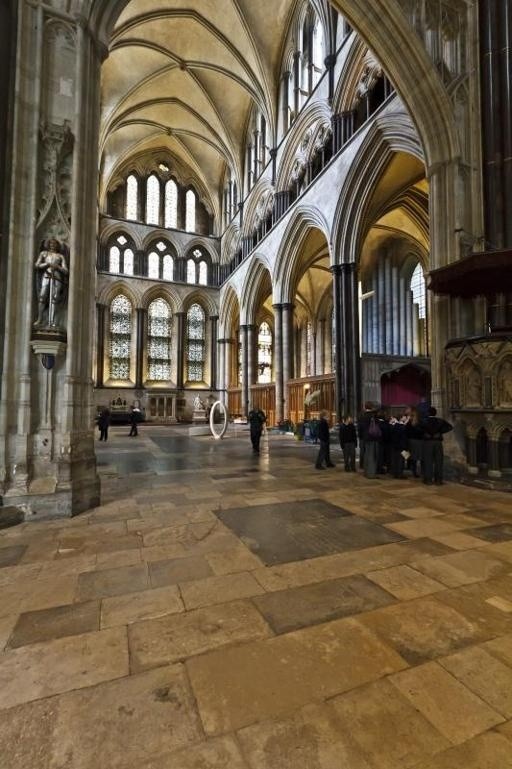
[248,402,266,456]
[96,407,111,443]
[314,410,335,470]
[359,400,453,486]
[340,414,358,472]
[33,239,68,327]
[127,405,141,437]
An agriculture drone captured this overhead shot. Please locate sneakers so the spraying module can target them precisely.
[326,463,337,467]
[315,466,327,470]
